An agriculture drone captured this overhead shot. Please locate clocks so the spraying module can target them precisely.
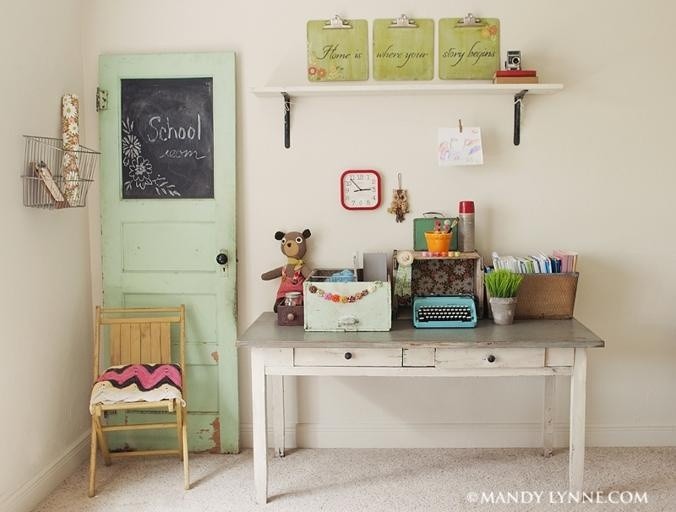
[341,170,382,210]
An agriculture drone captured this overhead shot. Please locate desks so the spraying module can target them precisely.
[235,312,606,499]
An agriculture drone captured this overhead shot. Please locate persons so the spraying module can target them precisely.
[260,229,314,314]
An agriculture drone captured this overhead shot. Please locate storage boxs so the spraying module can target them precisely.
[302,268,392,332]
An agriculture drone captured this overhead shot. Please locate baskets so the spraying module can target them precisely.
[484,266,579,320]
[20,135,102,209]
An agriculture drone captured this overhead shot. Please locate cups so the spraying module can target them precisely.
[423,230,453,256]
[488,296,519,325]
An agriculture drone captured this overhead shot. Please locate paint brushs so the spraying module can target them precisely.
[435,217,460,234]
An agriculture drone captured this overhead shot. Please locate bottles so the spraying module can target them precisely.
[458,200,476,253]
[284,291,302,307]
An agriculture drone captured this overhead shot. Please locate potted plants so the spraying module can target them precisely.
[483,266,524,325]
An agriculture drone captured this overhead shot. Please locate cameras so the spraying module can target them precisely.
[505,50,522,71]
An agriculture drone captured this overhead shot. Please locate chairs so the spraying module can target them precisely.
[87,304,189,498]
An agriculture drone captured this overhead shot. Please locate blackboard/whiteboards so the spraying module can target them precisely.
[120,77,214,199]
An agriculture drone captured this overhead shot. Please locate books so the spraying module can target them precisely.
[492,70,536,79]
[483,247,579,274]
[493,76,538,84]
